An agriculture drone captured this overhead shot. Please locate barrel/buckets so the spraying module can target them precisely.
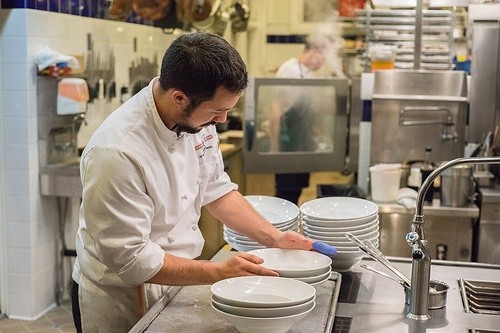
[369,163,403,204]
[439,161,475,208]
[368,46,397,70]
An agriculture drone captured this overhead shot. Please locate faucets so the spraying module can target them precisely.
[406,157,500,333]
[71,114,85,159]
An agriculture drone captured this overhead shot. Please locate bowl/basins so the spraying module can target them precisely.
[209,195,380,333]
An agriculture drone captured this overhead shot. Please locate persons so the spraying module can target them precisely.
[72,33,336,333]
[269,33,332,207]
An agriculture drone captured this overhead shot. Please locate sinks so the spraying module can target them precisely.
[40,158,83,198]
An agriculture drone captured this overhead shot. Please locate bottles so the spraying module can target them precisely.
[418,147,434,206]
